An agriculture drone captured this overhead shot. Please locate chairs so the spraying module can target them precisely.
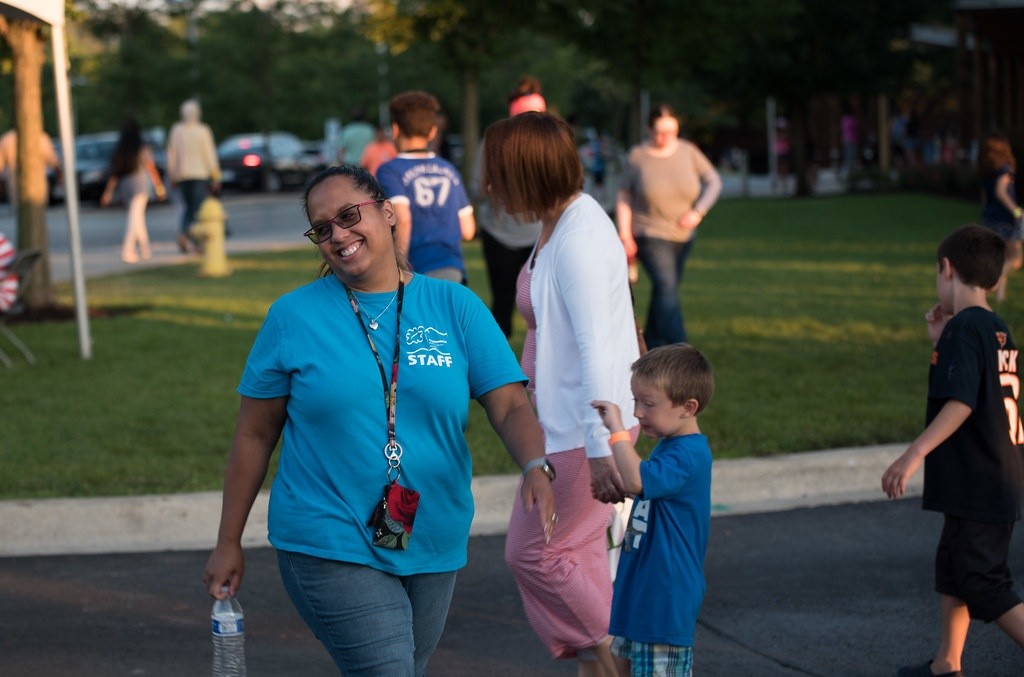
[0,248,44,372]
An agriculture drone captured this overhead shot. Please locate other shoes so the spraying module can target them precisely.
[177,236,188,254]
[121,250,154,263]
[898,659,964,677]
[194,243,202,253]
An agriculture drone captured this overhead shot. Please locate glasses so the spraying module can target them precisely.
[303,199,384,244]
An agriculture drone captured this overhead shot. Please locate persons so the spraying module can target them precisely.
[616,106,723,350]
[474,77,551,339]
[332,91,477,286]
[590,340,713,677]
[977,140,1024,303]
[882,223,1023,677]
[485,108,649,677]
[723,90,963,212]
[0,106,60,215]
[164,100,223,254]
[204,162,557,677]
[102,109,169,265]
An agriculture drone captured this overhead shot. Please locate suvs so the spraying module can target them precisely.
[57,133,166,205]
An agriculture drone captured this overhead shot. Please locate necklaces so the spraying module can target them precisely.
[351,288,398,331]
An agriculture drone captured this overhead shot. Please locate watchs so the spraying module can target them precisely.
[522,456,557,482]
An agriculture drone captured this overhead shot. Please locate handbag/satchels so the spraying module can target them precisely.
[205,185,234,236]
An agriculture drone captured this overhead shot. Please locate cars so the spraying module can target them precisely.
[571,126,604,186]
[215,131,315,194]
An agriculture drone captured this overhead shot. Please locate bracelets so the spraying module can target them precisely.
[608,430,630,447]
[1012,206,1024,218]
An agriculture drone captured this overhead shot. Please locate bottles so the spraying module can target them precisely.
[209,586,247,677]
